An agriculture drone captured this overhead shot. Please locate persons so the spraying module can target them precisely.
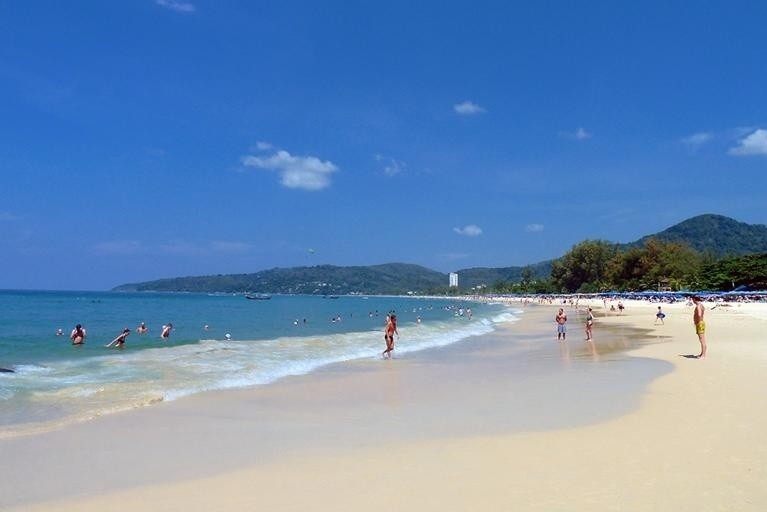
[585,307,595,342]
[103,328,130,348]
[56,328,64,336]
[70,323,87,345]
[135,322,148,334]
[693,295,708,360]
[555,307,567,340]
[381,315,400,363]
[160,323,173,338]
[655,305,666,326]
